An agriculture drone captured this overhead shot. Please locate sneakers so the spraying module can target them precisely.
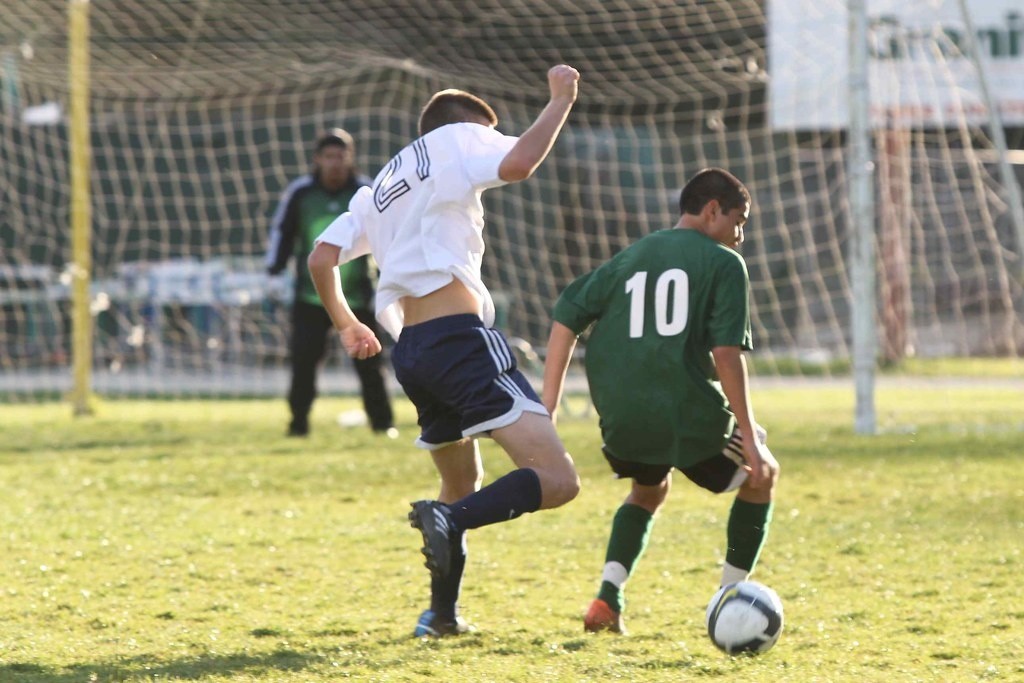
[413,609,479,639]
[408,499,461,582]
[583,599,631,636]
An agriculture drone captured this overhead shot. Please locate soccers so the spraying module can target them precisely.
[705,580,787,658]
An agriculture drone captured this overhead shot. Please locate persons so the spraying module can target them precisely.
[307,64,581,640]
[540,166,779,633]
[266,126,395,435]
